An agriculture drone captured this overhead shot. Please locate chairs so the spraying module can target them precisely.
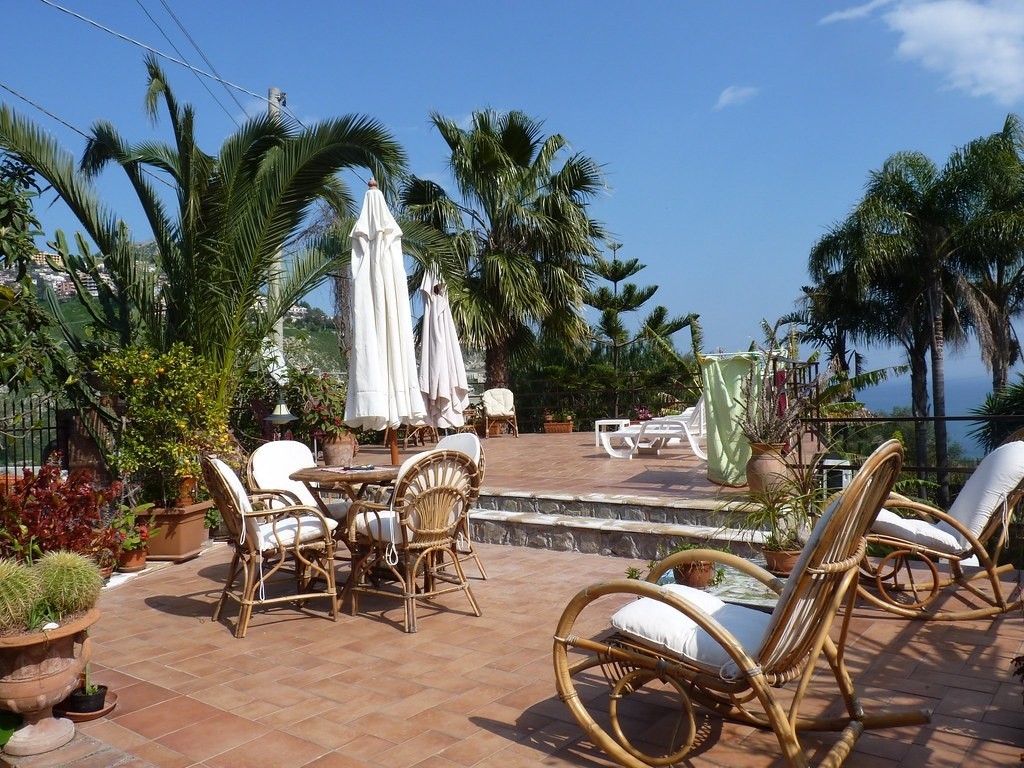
[347,448,483,634]
[482,388,519,439]
[374,433,488,578]
[816,428,1024,618]
[200,456,344,638]
[384,423,440,451]
[601,390,708,461]
[246,441,361,592]
[454,406,480,435]
[552,438,907,768]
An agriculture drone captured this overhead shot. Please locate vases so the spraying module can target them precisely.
[543,422,574,433]
[117,546,150,568]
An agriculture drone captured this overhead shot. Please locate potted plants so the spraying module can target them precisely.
[148,435,214,507]
[64,626,108,712]
[561,408,576,421]
[103,344,241,560]
[0,551,106,756]
[295,367,360,465]
[705,420,888,575]
[62,471,115,580]
[623,535,743,600]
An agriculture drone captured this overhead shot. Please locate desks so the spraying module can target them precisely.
[595,419,630,448]
[290,463,424,616]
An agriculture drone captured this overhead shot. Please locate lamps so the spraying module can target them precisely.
[263,400,299,440]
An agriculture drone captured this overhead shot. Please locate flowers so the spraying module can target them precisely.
[634,408,653,420]
[108,502,162,551]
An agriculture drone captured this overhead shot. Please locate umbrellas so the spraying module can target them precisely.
[419,259,470,437]
[345,177,427,465]
